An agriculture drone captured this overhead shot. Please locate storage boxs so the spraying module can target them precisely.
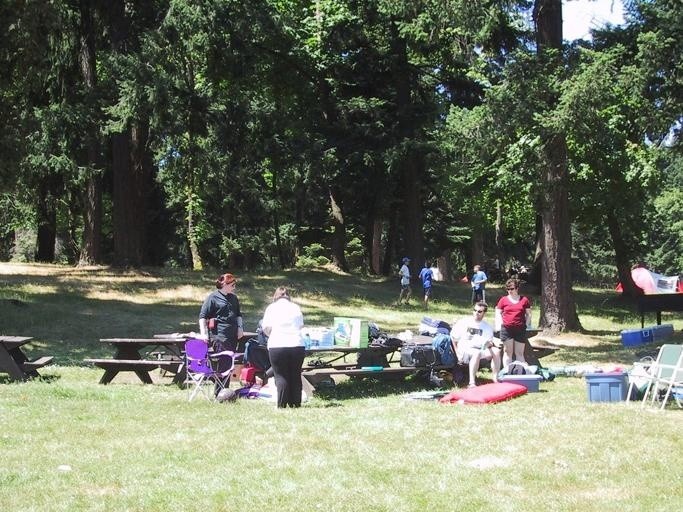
[501,375,541,393]
[620,323,651,345]
[584,371,628,402]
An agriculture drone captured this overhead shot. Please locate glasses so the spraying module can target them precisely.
[506,288,515,290]
[474,308,483,313]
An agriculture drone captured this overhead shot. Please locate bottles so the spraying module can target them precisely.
[304,334,310,350]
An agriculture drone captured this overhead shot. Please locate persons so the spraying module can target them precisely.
[262,287,306,409]
[397,258,412,306]
[471,264,487,305]
[495,278,532,367]
[449,301,500,389]
[419,259,434,310]
[199,273,244,397]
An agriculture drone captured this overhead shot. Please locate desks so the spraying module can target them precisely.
[308,329,550,370]
[100,338,185,384]
[146,331,258,363]
[0,336,53,381]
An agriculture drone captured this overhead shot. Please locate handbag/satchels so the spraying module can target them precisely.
[209,317,218,330]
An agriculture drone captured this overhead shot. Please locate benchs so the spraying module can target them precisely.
[296,349,553,391]
[81,359,186,384]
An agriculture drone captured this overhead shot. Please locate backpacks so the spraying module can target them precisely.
[434,334,458,368]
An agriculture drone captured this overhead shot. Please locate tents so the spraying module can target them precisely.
[615,268,683,294]
[656,345,683,382]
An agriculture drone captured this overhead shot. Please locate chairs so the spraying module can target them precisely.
[628,344,682,409]
[181,340,234,401]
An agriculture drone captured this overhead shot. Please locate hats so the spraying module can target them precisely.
[403,257,411,262]
